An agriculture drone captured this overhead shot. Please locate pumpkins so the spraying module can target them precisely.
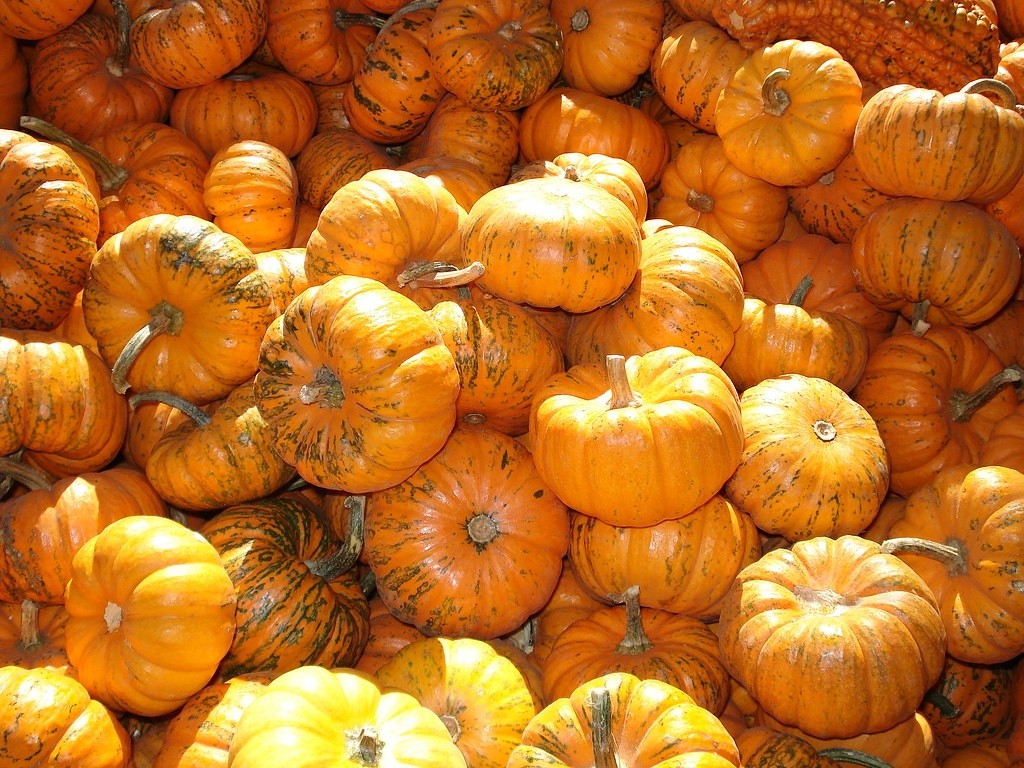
[0,0,1024,768]
